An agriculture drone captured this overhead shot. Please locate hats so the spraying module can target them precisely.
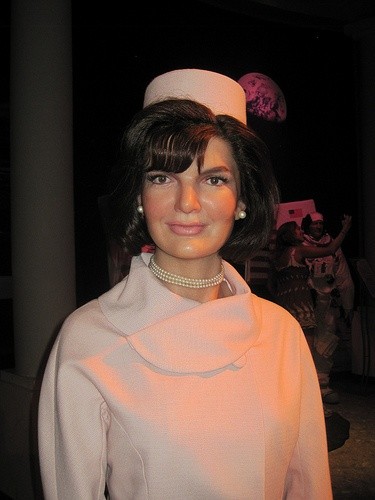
[300,212,324,229]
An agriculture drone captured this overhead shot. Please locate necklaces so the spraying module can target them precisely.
[148,254,224,289]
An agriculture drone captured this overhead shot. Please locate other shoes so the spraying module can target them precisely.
[323,392,339,417]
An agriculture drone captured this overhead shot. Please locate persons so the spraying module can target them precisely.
[246,211,375,418]
[36,68,333,500]
